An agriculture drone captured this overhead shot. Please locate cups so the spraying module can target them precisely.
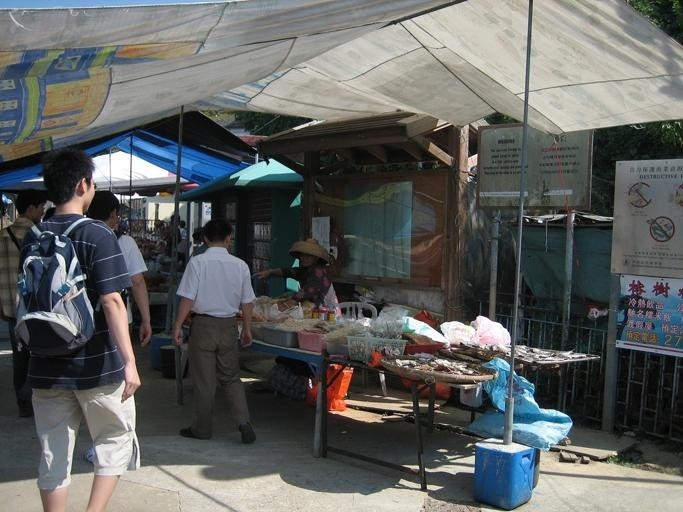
[310,306,336,321]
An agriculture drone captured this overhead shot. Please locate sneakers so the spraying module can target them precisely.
[239,421,256,443]
[179,426,211,438]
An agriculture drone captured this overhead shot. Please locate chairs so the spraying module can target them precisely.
[340,301,387,396]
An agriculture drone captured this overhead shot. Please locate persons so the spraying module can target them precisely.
[252,236,345,329]
[1,190,57,417]
[86,190,152,462]
[173,217,258,443]
[11,147,141,512]
[155,215,186,264]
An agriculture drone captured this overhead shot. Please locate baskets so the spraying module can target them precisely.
[347,336,408,362]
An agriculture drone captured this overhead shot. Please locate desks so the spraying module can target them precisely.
[174,324,601,490]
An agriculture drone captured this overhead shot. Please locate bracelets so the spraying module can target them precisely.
[282,301,289,308]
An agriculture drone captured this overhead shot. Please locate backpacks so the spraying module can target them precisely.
[12,217,105,358]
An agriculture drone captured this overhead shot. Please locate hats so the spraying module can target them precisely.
[287,238,328,262]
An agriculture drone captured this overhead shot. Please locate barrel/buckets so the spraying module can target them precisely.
[305,364,354,412]
[159,344,190,380]
[149,333,173,372]
